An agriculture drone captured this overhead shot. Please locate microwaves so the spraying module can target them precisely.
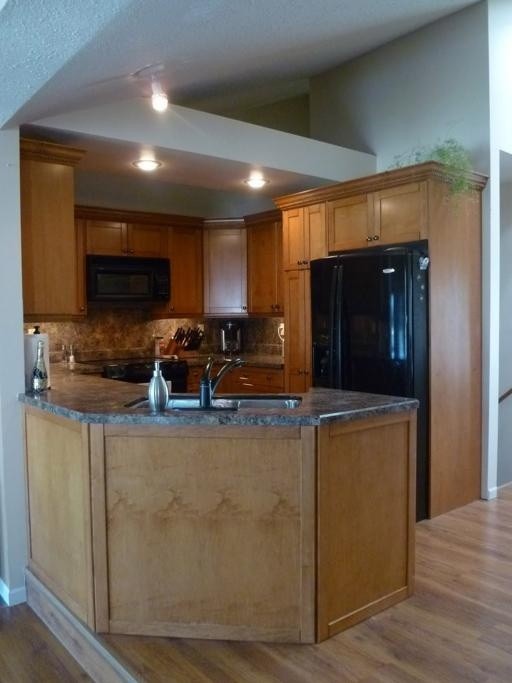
[86,254,172,301]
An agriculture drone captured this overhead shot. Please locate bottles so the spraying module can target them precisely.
[32,340,48,392]
[61,344,76,363]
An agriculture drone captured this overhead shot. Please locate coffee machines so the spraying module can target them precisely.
[220,321,242,356]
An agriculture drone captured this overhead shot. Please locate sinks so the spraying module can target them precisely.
[133,399,214,409]
[238,399,302,409]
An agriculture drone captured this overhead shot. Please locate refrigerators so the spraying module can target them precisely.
[309,235,431,520]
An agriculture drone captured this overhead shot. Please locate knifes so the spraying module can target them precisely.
[174,325,204,350]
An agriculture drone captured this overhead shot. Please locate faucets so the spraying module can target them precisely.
[199,357,245,408]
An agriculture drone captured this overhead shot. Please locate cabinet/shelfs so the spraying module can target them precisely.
[183,363,226,396]
[162,213,203,318]
[247,207,278,315]
[200,216,250,317]
[272,182,326,399]
[324,163,488,250]
[23,138,86,321]
[227,367,285,396]
[75,207,167,260]
